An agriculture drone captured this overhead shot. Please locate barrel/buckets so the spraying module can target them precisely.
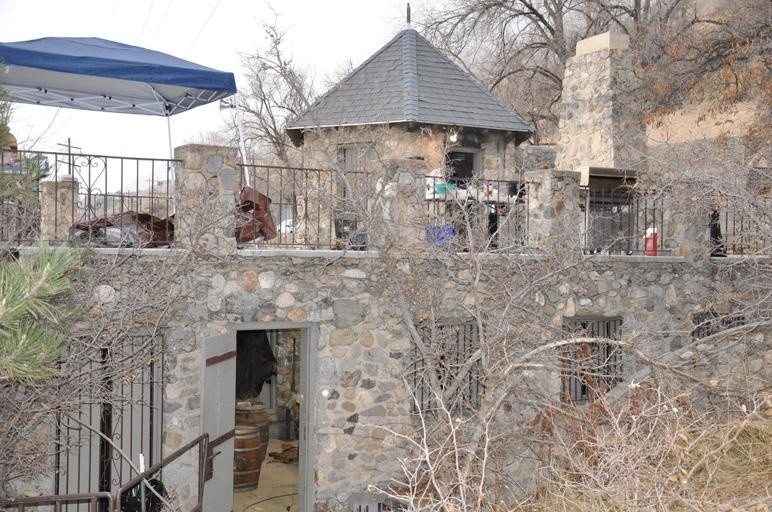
[233,425,264,494]
[234,400,271,463]
